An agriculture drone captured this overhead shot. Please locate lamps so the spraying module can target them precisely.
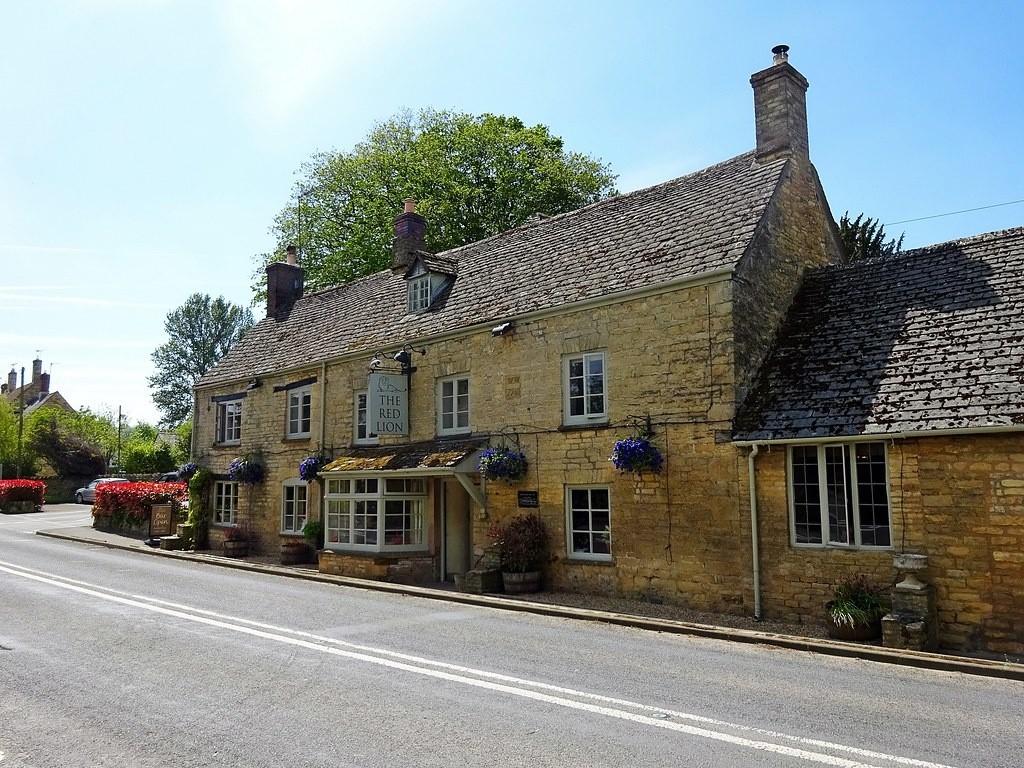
[247,378,264,391]
[393,343,427,362]
[370,350,407,368]
[491,322,513,338]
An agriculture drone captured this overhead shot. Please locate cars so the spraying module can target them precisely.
[75,478,130,504]
[156,472,184,483]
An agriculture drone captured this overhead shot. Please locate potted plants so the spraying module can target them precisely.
[281,534,306,565]
[221,522,252,559]
[826,573,888,644]
[485,512,554,597]
[604,525,610,553]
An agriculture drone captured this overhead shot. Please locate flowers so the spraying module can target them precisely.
[300,454,332,483]
[478,443,529,485]
[229,457,265,484]
[607,436,663,476]
[177,462,199,482]
